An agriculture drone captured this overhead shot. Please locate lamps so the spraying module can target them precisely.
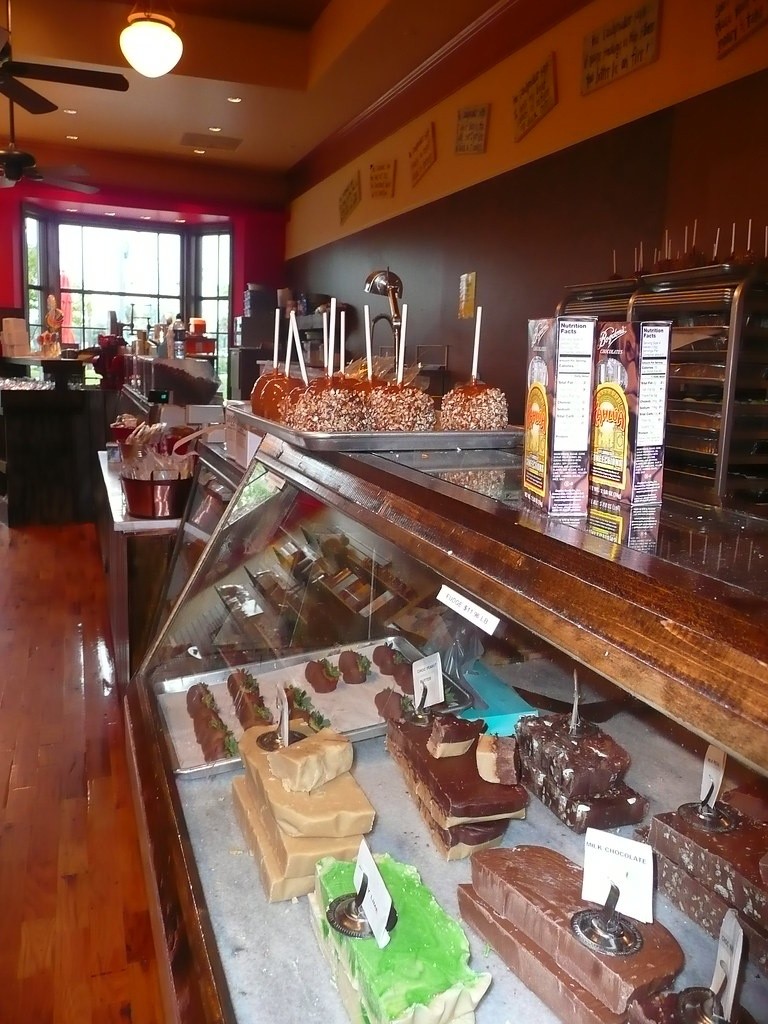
[0,0,44,189]
[118,0,183,78]
[363,264,404,375]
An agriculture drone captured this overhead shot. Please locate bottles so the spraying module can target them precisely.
[172,314,186,360]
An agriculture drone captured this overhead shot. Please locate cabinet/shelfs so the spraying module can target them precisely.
[124,431,768,1024]
[142,439,249,650]
[0,388,119,528]
[626,265,768,500]
[96,449,181,700]
[555,279,639,321]
[229,345,272,400]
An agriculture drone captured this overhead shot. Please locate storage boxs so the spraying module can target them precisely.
[244,289,271,318]
[295,313,347,368]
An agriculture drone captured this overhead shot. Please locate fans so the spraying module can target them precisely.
[0,25,130,115]
[26,163,100,194]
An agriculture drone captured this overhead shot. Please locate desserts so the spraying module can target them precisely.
[252,372,507,433]
[186,643,768,1024]
